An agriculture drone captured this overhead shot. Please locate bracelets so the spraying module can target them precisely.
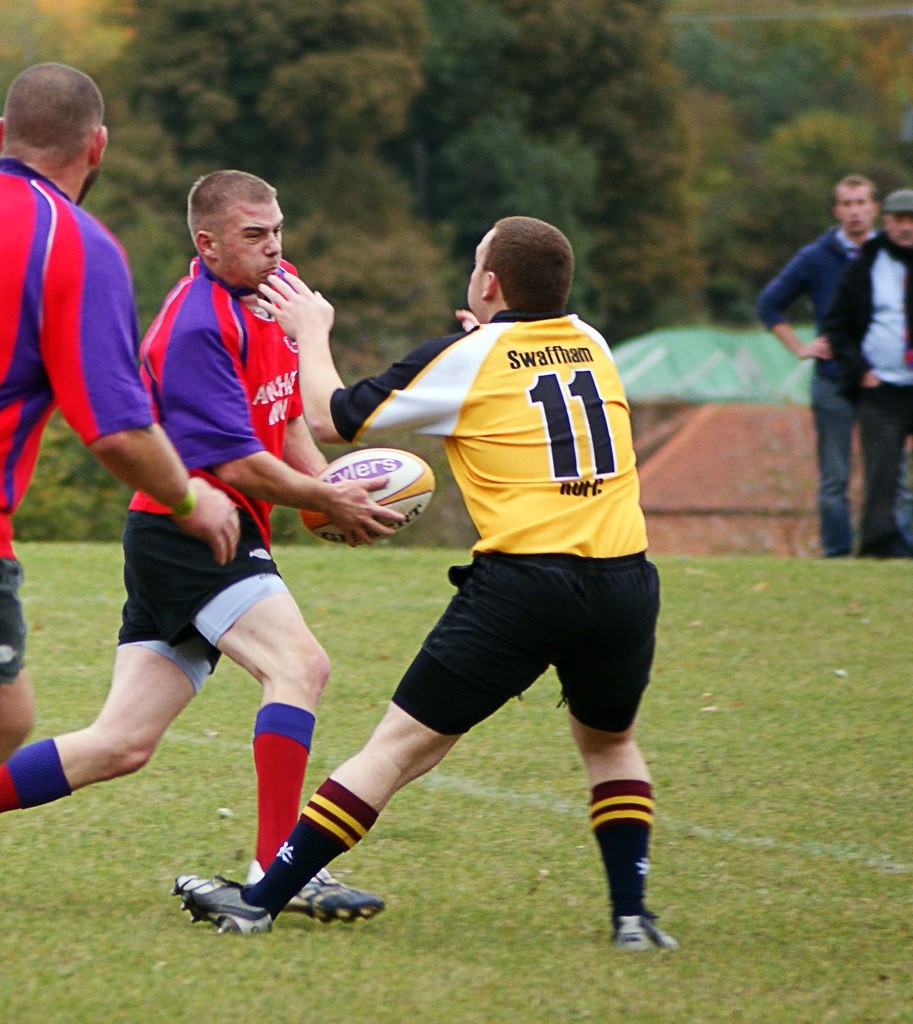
[172,482,195,517]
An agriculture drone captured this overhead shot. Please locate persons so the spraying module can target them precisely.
[0,169,406,925]
[0,60,240,765]
[172,216,681,951]
[758,173,913,559]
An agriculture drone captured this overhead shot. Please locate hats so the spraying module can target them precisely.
[881,188,913,213]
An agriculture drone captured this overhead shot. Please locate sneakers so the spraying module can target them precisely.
[247,860,385,924]
[169,875,273,938]
[612,916,680,955]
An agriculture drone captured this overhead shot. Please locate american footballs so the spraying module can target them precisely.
[300,448,435,544]
